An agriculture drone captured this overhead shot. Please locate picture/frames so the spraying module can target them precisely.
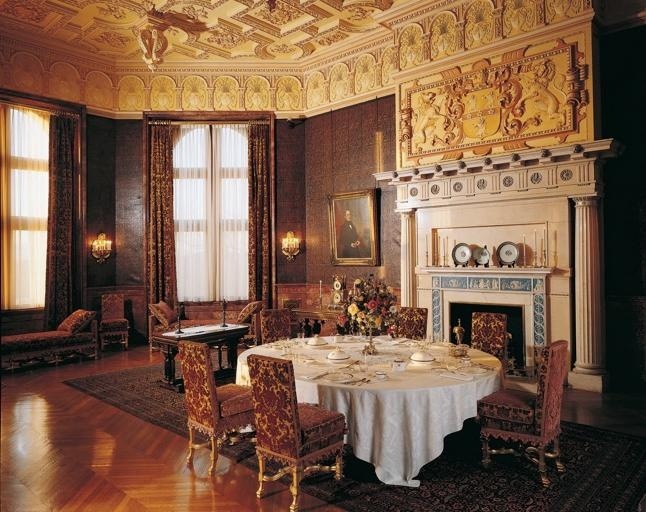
[326,188,381,267]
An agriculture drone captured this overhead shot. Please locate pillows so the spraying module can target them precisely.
[237,301,262,324]
[148,301,178,330]
[57,309,97,335]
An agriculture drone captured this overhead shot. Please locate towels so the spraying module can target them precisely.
[296,369,327,381]
[439,371,474,381]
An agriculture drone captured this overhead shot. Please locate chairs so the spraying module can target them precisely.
[282,299,302,337]
[260,308,291,344]
[398,307,428,339]
[478,340,568,489]
[178,341,254,477]
[100,293,128,351]
[247,354,345,512]
[471,312,512,375]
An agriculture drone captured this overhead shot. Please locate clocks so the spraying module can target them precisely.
[328,268,346,305]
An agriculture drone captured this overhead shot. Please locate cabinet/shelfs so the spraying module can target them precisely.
[292,310,343,338]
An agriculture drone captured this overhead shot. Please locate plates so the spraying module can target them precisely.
[302,333,328,349]
[409,351,437,366]
[472,246,491,265]
[496,241,521,265]
[454,365,488,375]
[451,243,471,266]
[325,345,351,365]
[320,372,353,384]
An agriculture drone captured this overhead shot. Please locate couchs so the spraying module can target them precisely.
[0,307,99,371]
[148,301,263,352]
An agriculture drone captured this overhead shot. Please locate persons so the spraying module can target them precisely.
[340,208,362,258]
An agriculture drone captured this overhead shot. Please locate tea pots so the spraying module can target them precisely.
[392,357,408,372]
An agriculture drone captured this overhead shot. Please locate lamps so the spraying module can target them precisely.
[281,230,300,263]
[91,233,113,263]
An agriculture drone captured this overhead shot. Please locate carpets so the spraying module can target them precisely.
[62,351,645,512]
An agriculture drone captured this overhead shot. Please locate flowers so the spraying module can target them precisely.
[338,277,401,356]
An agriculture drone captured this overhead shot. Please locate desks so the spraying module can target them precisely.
[153,324,249,392]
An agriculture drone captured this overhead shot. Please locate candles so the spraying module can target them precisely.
[320,280,322,296]
[534,230,536,250]
[523,234,526,256]
[354,282,356,297]
[435,233,438,255]
[443,237,445,256]
[425,233,428,251]
[543,229,546,250]
